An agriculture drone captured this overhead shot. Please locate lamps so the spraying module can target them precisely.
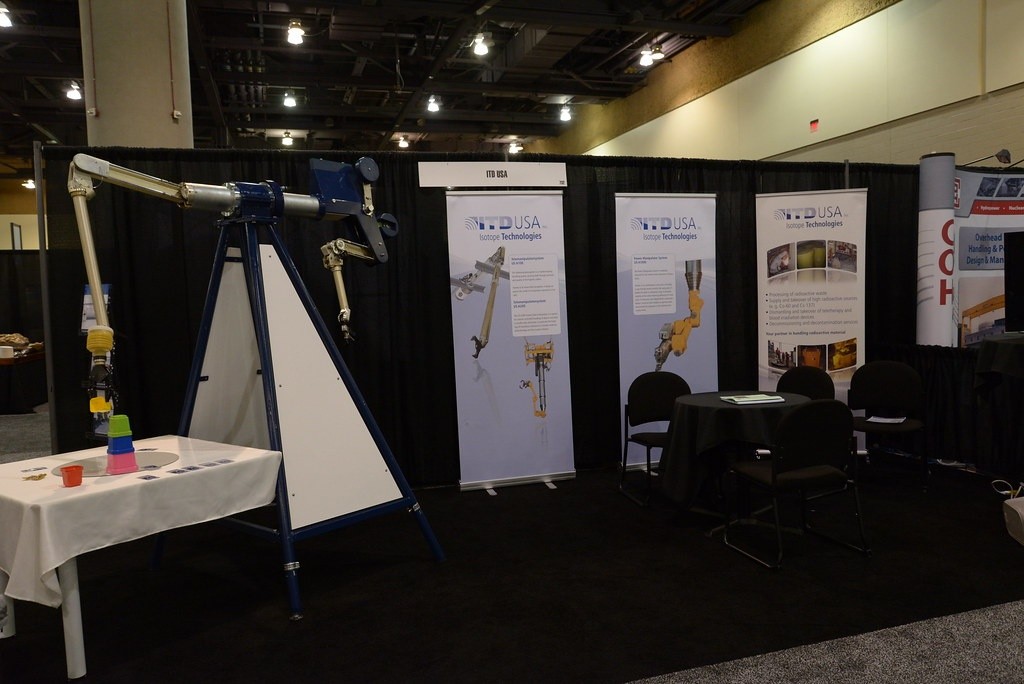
[286,18,306,45]
[651,34,665,60]
[474,31,494,55]
[399,135,410,148]
[640,43,653,67]
[21,179,35,188]
[560,99,572,121]
[509,142,524,153]
[0,1,13,28]
[427,95,442,111]
[281,132,293,145]
[67,81,82,100]
[284,89,297,107]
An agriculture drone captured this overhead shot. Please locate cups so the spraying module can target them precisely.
[106,415,139,475]
[0,346,13,358]
[60,465,84,486]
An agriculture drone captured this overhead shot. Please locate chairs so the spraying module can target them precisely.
[776,365,835,401]
[722,398,871,569]
[848,360,930,487]
[617,372,691,510]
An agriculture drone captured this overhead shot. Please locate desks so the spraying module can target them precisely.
[656,391,812,539]
